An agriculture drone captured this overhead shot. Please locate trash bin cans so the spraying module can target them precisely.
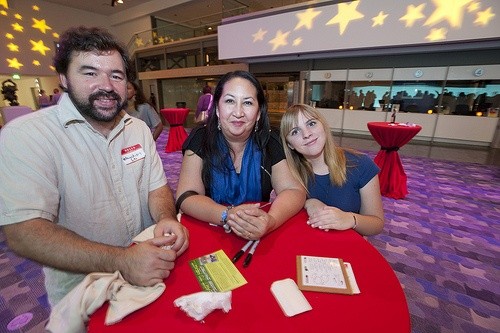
[176,102,185,108]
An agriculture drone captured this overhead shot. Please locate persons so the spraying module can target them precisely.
[175,70,306,240]
[52,88,61,104]
[194,86,214,128]
[349,89,476,116]
[38,89,49,103]
[122,70,163,143]
[0,25,189,313]
[280,103,384,235]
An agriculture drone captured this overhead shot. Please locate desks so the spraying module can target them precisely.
[86,201,412,333]
[367,122,422,199]
[160,108,190,152]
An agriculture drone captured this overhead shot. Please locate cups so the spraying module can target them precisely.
[488,108,500,118]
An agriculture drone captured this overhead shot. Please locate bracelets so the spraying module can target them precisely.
[222,206,231,234]
[352,212,357,230]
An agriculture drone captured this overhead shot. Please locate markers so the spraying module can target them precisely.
[234,240,253,262]
[243,239,260,266]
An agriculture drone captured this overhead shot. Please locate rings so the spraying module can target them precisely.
[247,233,250,237]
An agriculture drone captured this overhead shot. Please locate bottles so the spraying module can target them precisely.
[392,112,395,123]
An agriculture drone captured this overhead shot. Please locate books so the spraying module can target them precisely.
[190,249,247,293]
